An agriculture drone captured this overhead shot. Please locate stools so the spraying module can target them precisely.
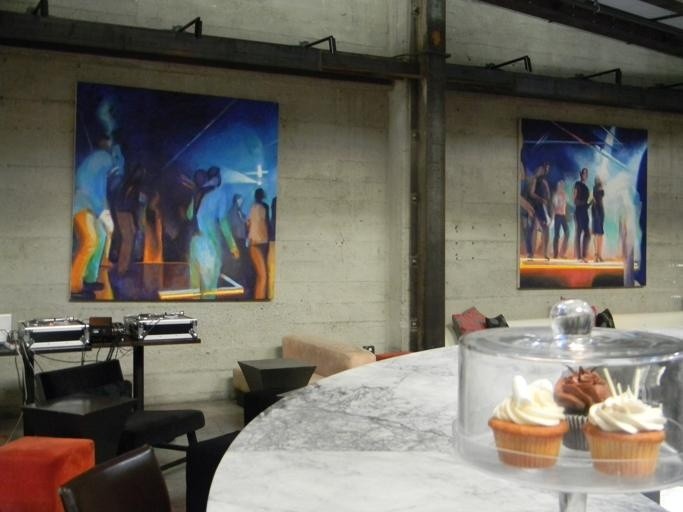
[0,436,94,512]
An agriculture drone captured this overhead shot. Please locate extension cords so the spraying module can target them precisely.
[6,341,29,350]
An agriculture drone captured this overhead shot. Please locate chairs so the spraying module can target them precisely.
[20,358,205,512]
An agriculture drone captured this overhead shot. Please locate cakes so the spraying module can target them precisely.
[584,386,666,481]
[489,374,569,469]
[555,367,613,454]
